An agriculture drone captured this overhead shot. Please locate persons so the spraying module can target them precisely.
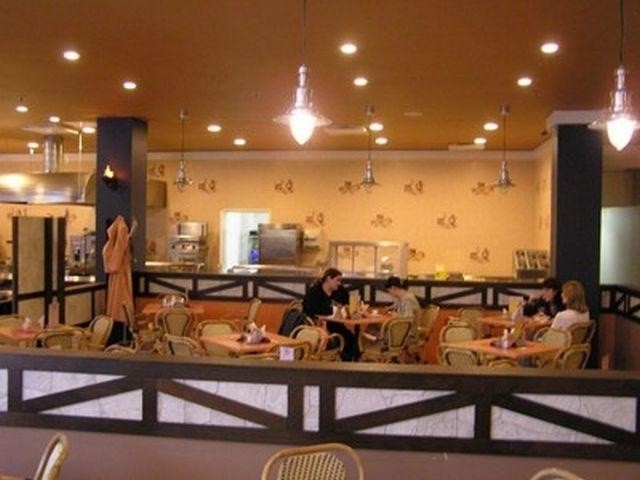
[521,275,566,321]
[300,267,361,362]
[548,280,591,335]
[381,276,423,316]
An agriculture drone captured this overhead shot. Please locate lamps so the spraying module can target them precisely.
[588,2,639,153]
[270,2,335,149]
[489,103,519,191]
[356,103,381,190]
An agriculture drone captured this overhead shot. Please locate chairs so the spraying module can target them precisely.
[0,292,597,369]
[261,443,366,480]
[0,432,69,480]
[533,467,584,480]
